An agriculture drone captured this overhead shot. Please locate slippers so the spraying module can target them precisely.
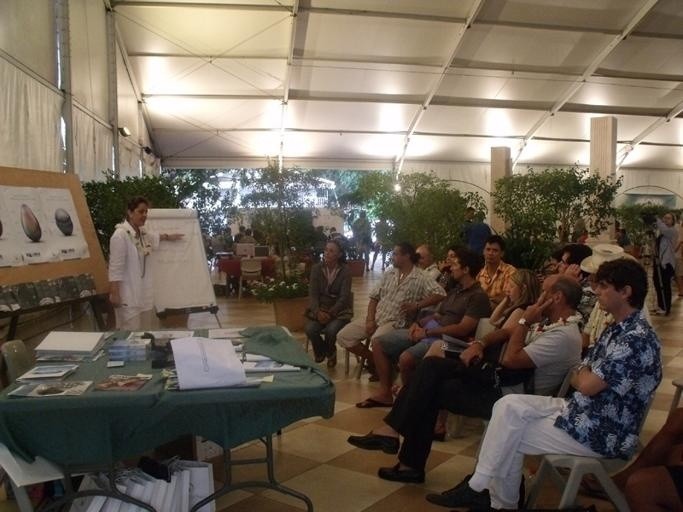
[355,397,394,409]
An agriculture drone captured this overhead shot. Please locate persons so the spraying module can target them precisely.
[108,197,185,330]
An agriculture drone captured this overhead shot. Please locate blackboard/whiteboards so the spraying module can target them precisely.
[129,210,219,319]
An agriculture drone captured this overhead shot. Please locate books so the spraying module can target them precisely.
[7,330,194,399]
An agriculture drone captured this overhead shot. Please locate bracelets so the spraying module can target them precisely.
[164,233,168,240]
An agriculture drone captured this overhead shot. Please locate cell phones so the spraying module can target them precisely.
[471,356,481,366]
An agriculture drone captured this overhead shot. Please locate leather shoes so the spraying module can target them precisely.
[377,462,426,486]
[345,430,400,456]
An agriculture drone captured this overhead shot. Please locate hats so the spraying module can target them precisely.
[579,243,640,275]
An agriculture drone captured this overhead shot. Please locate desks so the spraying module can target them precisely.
[0,324,337,512]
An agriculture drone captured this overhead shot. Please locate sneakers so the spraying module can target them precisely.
[313,345,337,369]
[424,474,491,511]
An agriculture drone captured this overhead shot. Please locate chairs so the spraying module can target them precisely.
[474,360,582,460]
[445,318,497,438]
[0,336,33,389]
[306,292,361,374]
[209,242,368,301]
[356,336,399,380]
[524,391,656,511]
